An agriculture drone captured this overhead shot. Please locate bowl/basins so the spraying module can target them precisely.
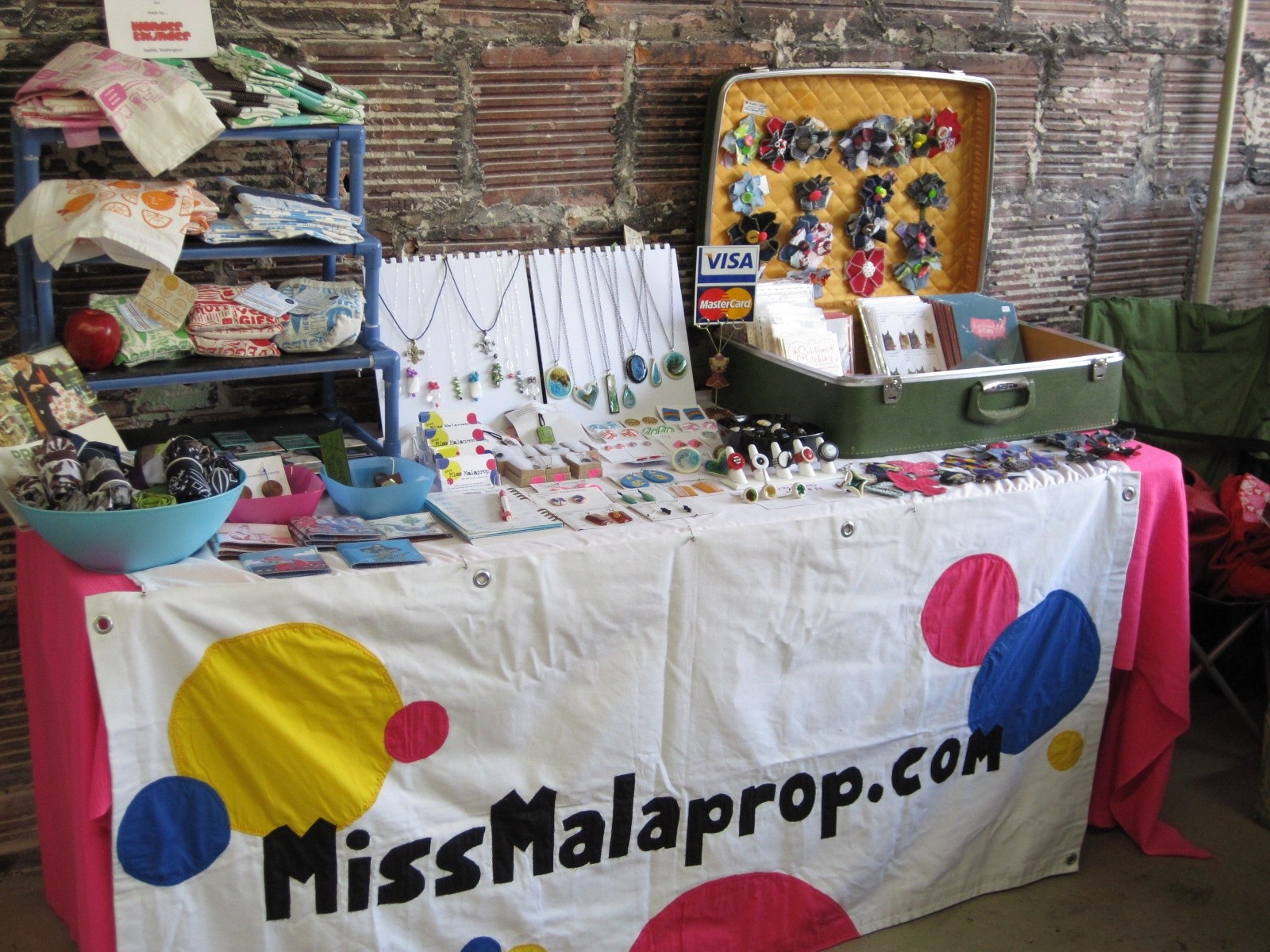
[226,465,325,525]
[7,466,245,572]
[320,456,436,520]
[716,413,825,466]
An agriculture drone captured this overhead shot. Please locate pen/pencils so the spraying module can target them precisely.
[498,490,512,522]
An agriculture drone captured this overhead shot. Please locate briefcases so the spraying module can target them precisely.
[688,62,1127,458]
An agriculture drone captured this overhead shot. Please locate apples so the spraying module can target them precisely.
[64,308,120,370]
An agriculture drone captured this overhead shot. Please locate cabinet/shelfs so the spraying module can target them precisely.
[9,116,401,468]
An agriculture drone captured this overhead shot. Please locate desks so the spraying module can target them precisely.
[13,428,1209,952]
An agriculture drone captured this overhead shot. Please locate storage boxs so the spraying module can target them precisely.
[496,440,603,489]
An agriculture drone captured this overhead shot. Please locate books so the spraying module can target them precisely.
[919,290,1027,369]
[424,483,566,546]
[857,293,946,376]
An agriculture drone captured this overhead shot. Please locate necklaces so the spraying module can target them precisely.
[593,251,648,383]
[623,249,662,387]
[554,251,600,410]
[603,251,637,409]
[584,252,620,414]
[447,252,523,355]
[377,259,446,365]
[633,249,688,380]
[531,250,573,399]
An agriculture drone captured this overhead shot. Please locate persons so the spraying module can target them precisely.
[706,354,729,389]
[1,353,66,434]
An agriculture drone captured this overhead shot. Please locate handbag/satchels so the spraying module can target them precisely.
[1181,466,1270,604]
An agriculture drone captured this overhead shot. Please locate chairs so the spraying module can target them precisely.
[1083,295,1270,742]
